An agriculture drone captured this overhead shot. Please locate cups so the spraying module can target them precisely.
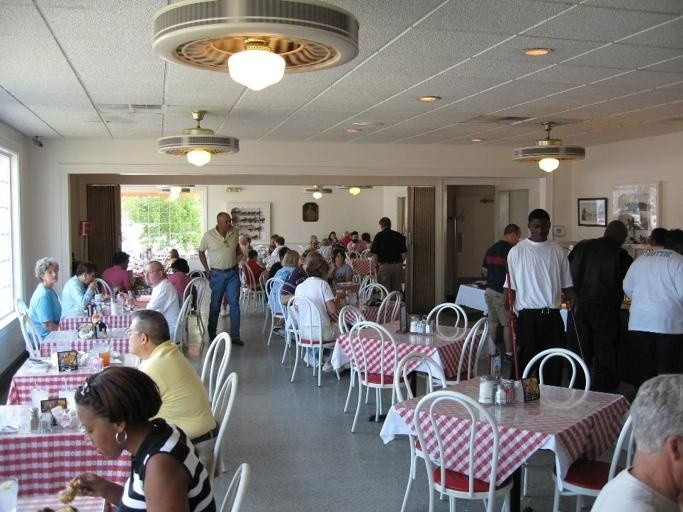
[0,475,17,511]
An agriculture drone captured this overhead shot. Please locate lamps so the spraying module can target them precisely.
[304,185,332,199]
[152,0,359,91]
[161,188,191,195]
[512,121,585,173]
[158,110,239,168]
[338,186,373,195]
[79,221,92,262]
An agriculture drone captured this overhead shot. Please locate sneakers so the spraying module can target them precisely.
[273,328,285,337]
[303,352,332,373]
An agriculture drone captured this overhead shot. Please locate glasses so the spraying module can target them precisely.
[80,379,101,407]
[125,329,144,336]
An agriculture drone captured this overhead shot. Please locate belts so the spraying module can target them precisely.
[212,264,237,271]
[190,423,219,444]
[524,309,559,314]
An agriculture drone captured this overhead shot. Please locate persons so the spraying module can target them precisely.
[126,310,220,478]
[199,212,244,347]
[101,252,133,296]
[126,261,181,342]
[165,249,191,304]
[62,261,109,317]
[480,209,683,392]
[591,374,683,512]
[71,367,216,512]
[240,217,407,373]
[28,257,62,348]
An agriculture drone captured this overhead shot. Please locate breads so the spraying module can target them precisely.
[56,506,76,512]
[58,481,78,504]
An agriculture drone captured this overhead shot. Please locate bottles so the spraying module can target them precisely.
[406,312,434,334]
[27,283,133,436]
[478,373,524,408]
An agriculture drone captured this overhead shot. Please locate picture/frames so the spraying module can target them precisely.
[303,203,319,222]
[578,197,607,227]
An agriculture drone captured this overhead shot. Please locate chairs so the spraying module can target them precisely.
[520,348,591,497]
[392,352,450,512]
[198,332,233,472]
[286,296,342,386]
[551,414,630,512]
[348,321,411,432]
[240,244,631,355]
[210,371,238,482]
[414,390,514,511]
[219,463,248,509]
[0,257,240,352]
[426,316,489,394]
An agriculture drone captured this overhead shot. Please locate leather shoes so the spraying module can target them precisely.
[232,340,243,345]
